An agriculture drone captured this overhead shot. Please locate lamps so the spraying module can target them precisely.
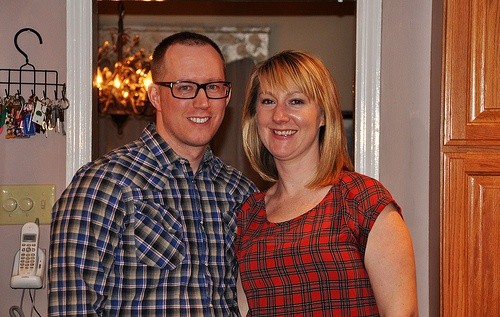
[93,1,155,117]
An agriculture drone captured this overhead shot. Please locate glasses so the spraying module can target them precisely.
[154,81,233,99]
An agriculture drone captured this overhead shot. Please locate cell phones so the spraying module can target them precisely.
[18,218,40,277]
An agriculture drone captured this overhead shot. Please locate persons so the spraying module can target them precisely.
[235,49,420,317]
[46,30,260,317]
